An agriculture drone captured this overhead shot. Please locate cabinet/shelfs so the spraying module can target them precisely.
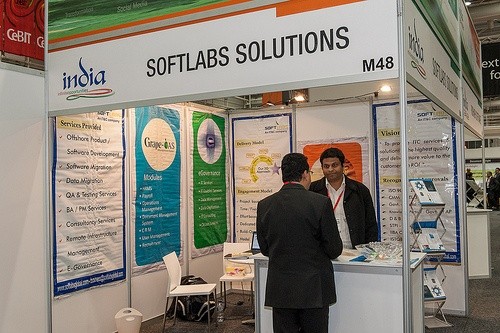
[409,193,452,329]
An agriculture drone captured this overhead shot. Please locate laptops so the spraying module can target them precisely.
[244,232,260,255]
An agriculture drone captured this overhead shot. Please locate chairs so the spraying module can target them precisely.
[218,242,255,313]
[163,251,218,333]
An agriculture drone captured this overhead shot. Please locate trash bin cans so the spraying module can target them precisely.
[114,307,143,333]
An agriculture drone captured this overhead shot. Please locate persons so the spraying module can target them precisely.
[309,147,379,251]
[486,167,500,210]
[255,153,343,332]
[466,168,474,179]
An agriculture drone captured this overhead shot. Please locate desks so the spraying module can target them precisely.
[248,251,427,333]
[467,206,492,279]
